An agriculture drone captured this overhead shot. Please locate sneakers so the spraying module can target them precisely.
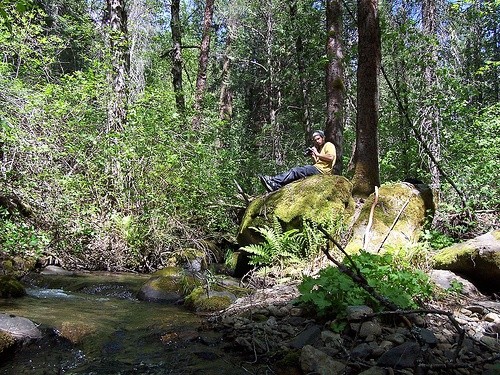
[260,176,274,192]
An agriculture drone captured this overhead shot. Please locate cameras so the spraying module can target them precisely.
[304,147,313,157]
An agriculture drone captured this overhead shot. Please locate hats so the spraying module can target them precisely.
[313,130,324,137]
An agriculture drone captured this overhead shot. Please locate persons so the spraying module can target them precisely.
[259,130,336,191]
